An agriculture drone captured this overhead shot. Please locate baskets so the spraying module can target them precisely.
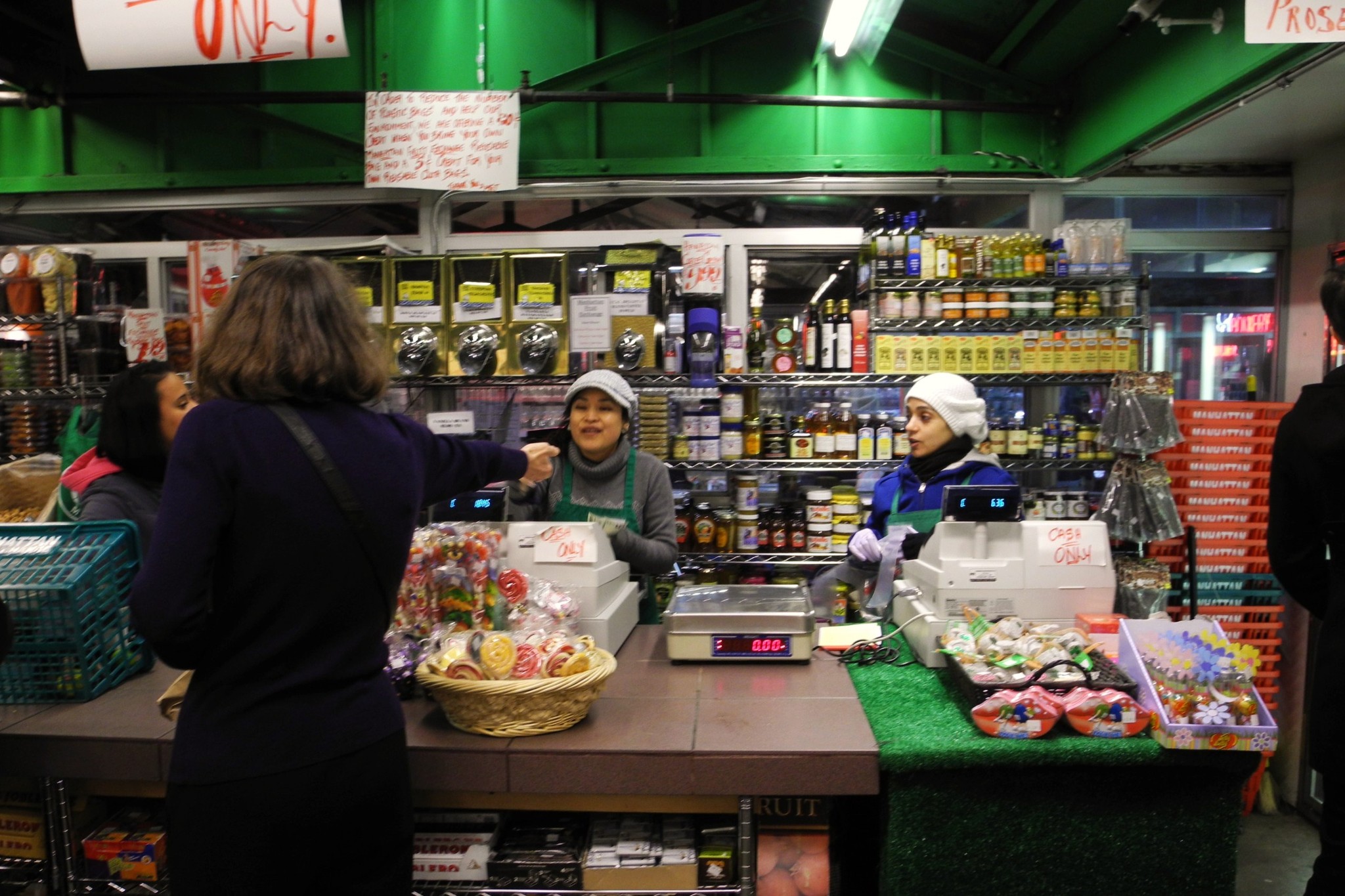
[1153,400,1297,812]
[413,646,617,736]
[1,520,155,704]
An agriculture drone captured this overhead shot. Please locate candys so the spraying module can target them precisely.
[384,527,590,686]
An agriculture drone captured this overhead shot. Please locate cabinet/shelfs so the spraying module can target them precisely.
[0,240,1167,590]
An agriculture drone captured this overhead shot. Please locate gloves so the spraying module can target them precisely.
[848,528,882,563]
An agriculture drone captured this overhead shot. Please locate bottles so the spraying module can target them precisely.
[674,387,1116,463]
[652,489,735,625]
[735,475,862,622]
[750,205,1141,373]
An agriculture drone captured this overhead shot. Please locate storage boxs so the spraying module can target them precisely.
[0,521,154,705]
[933,619,1137,705]
[488,818,586,889]
[580,820,699,891]
[1076,402,1289,813]
[82,812,166,883]
[698,828,737,887]
[756,824,831,896]
[412,811,502,880]
[0,789,94,861]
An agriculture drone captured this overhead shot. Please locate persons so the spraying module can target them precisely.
[1266,263,1345,896]
[507,369,678,625]
[127,253,560,896]
[76,359,200,552]
[811,372,1022,608]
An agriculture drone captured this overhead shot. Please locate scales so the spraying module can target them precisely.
[664,583,815,666]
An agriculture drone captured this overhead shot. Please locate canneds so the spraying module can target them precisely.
[879,282,1135,318]
[1044,492,1090,520]
[770,317,798,373]
[653,475,847,627]
[675,393,1120,460]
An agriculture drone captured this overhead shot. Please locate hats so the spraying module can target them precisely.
[564,368,639,415]
[905,372,989,440]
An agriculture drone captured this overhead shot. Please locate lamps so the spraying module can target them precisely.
[814,0,904,64]
